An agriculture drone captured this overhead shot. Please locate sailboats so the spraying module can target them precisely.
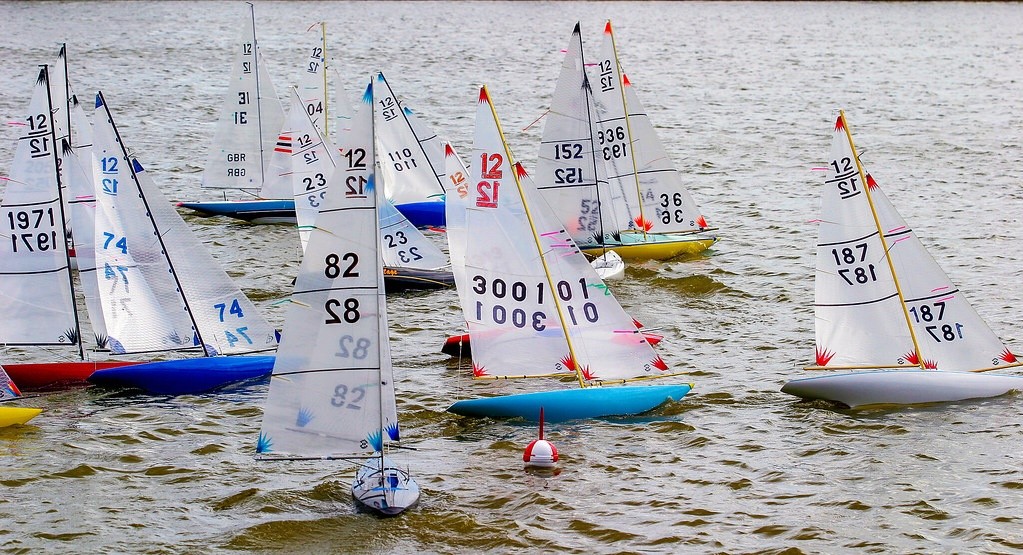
[442,139,663,356]
[535,19,718,259]
[781,107,1022,411]
[0,364,44,429]
[437,82,694,423]
[254,74,422,519]
[177,1,448,229]
[288,86,455,288]
[87,91,305,385]
[1,41,153,385]
